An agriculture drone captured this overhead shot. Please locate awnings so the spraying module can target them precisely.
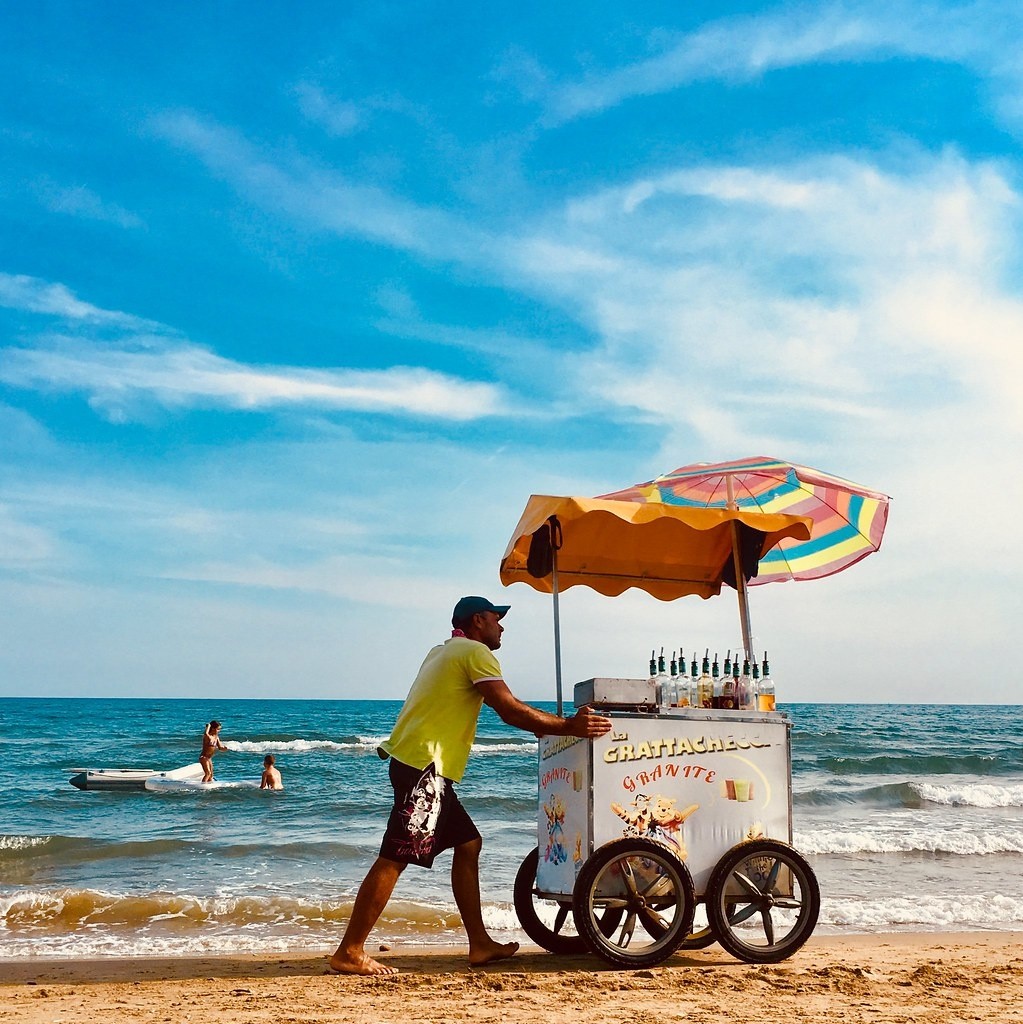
[498,492,814,601]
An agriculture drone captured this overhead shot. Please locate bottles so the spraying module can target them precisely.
[752,663,762,711]
[758,661,775,711]
[697,658,714,708]
[675,657,690,707]
[656,656,670,708]
[690,661,698,709]
[712,662,720,709]
[732,663,742,710]
[720,659,733,709]
[669,661,678,708]
[739,660,755,711]
[647,660,660,714]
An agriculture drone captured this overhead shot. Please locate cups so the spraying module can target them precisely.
[734,780,750,803]
[726,779,739,800]
[749,780,756,800]
[719,781,728,798]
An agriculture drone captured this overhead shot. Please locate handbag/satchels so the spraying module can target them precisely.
[527,514,566,580]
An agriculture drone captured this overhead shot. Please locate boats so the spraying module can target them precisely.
[144,776,268,793]
[64,762,215,793]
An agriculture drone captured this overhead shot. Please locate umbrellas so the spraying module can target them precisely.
[589,455,893,687]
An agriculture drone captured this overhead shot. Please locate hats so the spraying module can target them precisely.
[445,597,507,622]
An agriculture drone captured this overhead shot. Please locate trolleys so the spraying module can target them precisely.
[499,455,889,965]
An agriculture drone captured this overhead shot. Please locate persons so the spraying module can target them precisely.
[327,596,613,976]
[199,720,228,784]
[258,754,283,790]
